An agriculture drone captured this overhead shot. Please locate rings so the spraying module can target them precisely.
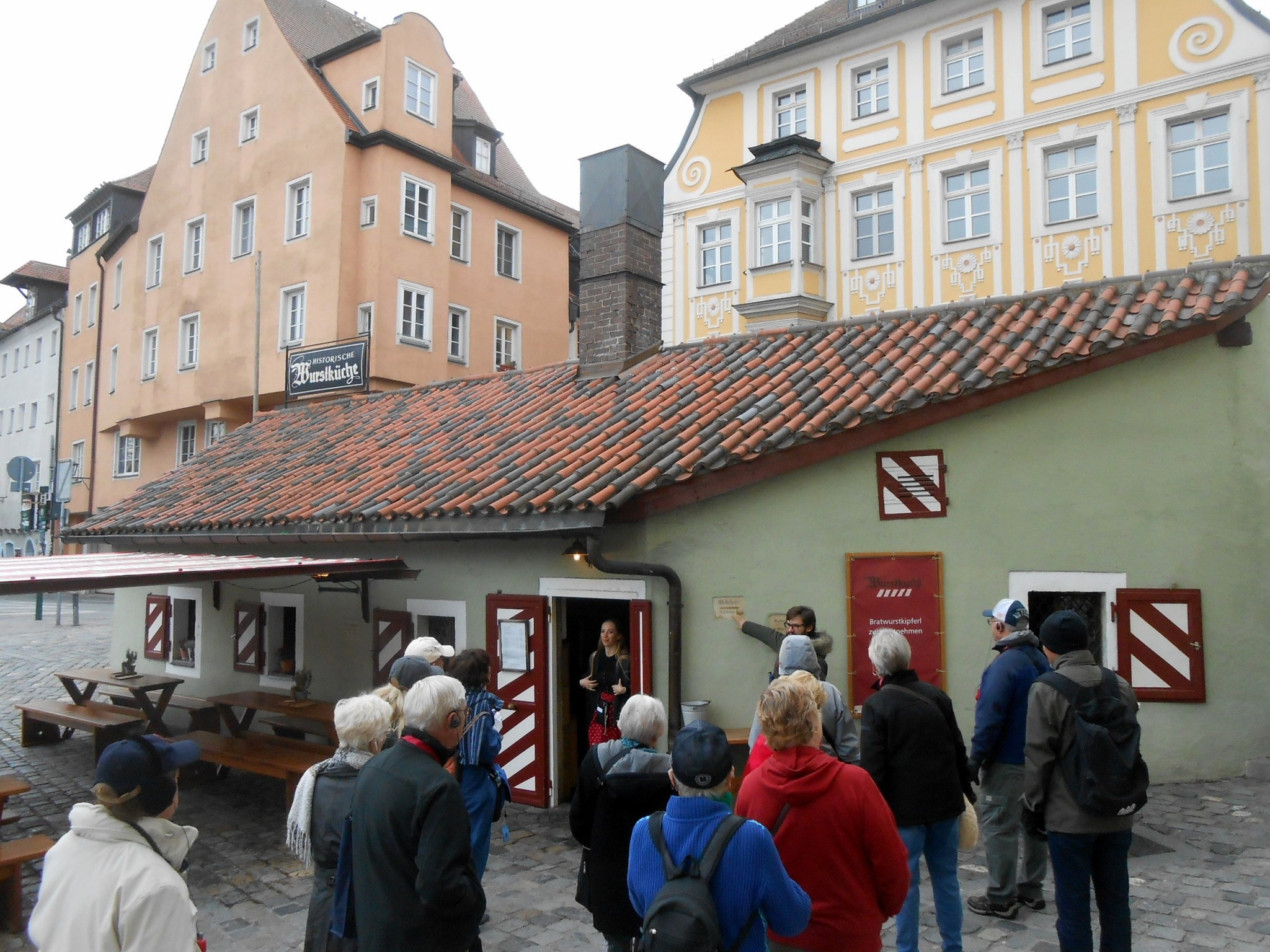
[590,685,592,689]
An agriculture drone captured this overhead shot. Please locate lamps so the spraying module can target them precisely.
[73,461,80,476]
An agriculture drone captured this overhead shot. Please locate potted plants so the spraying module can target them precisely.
[277,648,295,674]
[178,639,189,660]
[500,361,517,372]
[291,662,315,700]
[121,648,137,674]
[184,639,195,661]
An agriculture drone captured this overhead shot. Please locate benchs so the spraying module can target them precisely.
[1,832,57,936]
[0,774,32,828]
[258,714,340,747]
[98,688,222,734]
[15,701,145,769]
[172,729,331,810]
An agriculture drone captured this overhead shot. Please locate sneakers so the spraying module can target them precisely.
[1014,892,1046,909]
[968,894,1018,919]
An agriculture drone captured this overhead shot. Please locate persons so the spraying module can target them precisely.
[627,719,813,952]
[858,627,980,952]
[28,734,209,952]
[744,670,829,779]
[576,616,631,747]
[968,597,1056,921]
[363,655,461,786]
[437,648,513,924]
[746,634,861,769]
[568,692,680,952]
[284,695,395,951]
[731,676,913,952]
[1017,608,1151,952]
[403,635,456,677]
[733,605,836,683]
[343,674,490,952]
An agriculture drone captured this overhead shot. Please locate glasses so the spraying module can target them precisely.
[783,621,806,630]
[987,618,1001,626]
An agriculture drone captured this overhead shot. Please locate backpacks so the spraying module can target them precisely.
[628,809,747,952]
[1033,664,1151,818]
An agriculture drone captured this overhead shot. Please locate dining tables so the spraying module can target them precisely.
[54,666,186,740]
[205,689,336,777]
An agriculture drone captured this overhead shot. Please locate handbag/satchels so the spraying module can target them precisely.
[574,846,596,916]
[329,815,358,940]
[492,762,514,824]
[954,791,978,852]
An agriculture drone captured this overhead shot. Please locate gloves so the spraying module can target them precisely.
[964,784,977,806]
[1020,802,1049,843]
[965,755,983,786]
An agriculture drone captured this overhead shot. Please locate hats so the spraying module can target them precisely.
[93,733,201,804]
[1039,609,1088,655]
[671,718,735,791]
[404,636,456,664]
[983,598,1031,628]
[388,655,445,693]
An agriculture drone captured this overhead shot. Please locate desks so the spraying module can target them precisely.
[724,728,755,797]
